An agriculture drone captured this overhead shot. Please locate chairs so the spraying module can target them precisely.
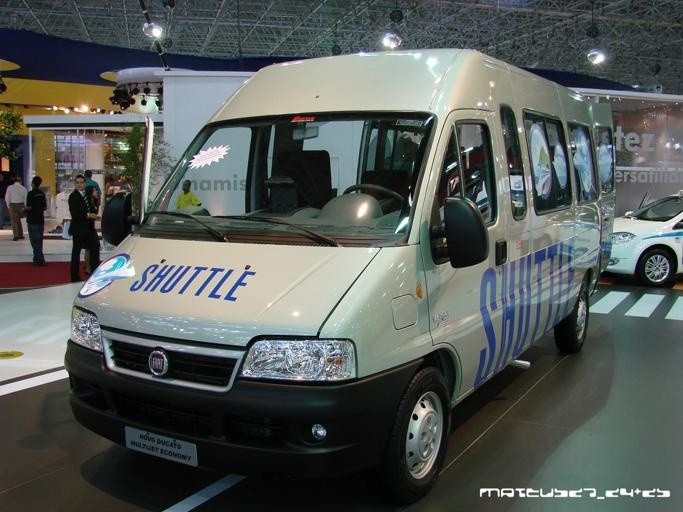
[278,149,332,208]
[358,169,408,214]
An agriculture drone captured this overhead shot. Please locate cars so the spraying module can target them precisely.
[609,194,682,286]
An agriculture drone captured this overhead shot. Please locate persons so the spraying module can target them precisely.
[0,174,7,230]
[5,177,28,240]
[68,170,132,282]
[27,176,47,266]
[177,180,201,210]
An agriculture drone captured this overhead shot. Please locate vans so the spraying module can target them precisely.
[65,48,613,500]
